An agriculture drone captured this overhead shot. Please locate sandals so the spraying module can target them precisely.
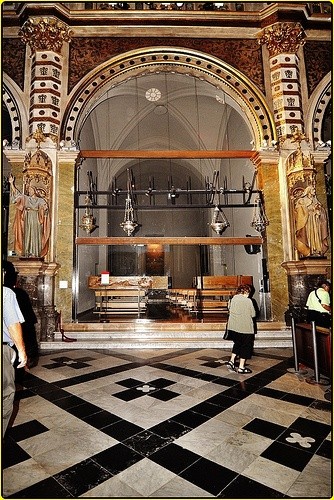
[238,367,252,374]
[226,361,237,373]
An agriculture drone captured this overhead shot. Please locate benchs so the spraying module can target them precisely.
[94,289,152,317]
[165,289,238,317]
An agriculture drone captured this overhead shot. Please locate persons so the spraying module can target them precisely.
[294,185,329,257]
[223,284,256,374]
[306,281,331,315]
[3,286,28,438]
[9,173,51,259]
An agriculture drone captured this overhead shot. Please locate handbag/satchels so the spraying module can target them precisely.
[223,329,233,340]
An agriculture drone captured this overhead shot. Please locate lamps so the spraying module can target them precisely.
[271,140,277,148]
[112,187,122,197]
[250,175,269,237]
[78,176,97,233]
[207,191,230,235]
[145,188,151,197]
[315,140,331,148]
[3,139,9,150]
[120,188,139,237]
[12,140,19,150]
[170,187,175,197]
[70,140,77,151]
[263,139,269,148]
[60,140,68,150]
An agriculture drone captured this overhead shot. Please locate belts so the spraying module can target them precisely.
[2,342,9,345]
